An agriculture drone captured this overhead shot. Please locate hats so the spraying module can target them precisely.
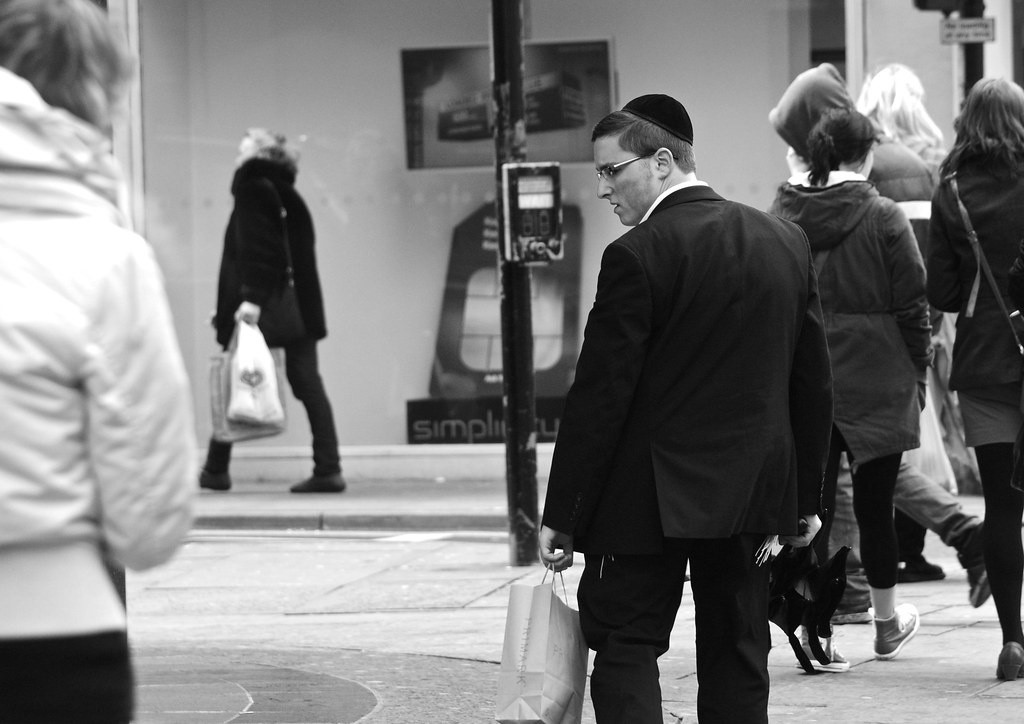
[620,93,694,145]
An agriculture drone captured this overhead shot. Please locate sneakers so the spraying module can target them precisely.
[796,621,853,673]
[956,523,991,607]
[873,602,920,660]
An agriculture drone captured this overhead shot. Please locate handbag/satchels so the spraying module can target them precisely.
[494,560,589,724]
[209,313,287,444]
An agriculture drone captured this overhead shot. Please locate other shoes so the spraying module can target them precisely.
[898,555,946,583]
[199,465,234,490]
[289,470,346,492]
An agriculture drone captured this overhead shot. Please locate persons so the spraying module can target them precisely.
[0,0,201,724]
[535,95,835,724]
[196,128,348,493]
[769,62,991,673]
[928,76,1024,680]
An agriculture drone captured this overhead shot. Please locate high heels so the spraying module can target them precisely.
[997,641,1024,681]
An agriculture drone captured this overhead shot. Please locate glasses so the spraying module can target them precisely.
[596,152,678,182]
